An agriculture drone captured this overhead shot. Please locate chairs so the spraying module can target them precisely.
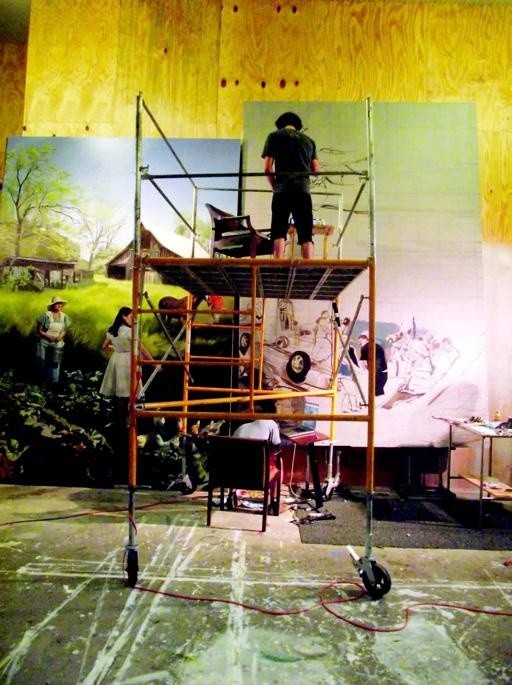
[209,438,277,531]
[205,201,275,258]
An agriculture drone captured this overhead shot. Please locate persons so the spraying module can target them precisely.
[260,111,320,259]
[295,311,450,410]
[226,404,291,515]
[99,306,163,401]
[35,296,69,383]
[205,295,224,324]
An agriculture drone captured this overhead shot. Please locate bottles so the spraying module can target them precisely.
[493,410,501,421]
[193,419,225,435]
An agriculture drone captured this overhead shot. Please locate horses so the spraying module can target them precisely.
[158,289,205,340]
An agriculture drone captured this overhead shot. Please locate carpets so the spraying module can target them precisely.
[292,484,511,549]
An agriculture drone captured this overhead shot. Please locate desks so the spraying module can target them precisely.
[282,425,330,513]
[285,222,333,258]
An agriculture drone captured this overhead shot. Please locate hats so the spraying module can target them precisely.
[48,296,68,311]
[359,331,368,339]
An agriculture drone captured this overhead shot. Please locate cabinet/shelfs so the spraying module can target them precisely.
[448,420,511,520]
